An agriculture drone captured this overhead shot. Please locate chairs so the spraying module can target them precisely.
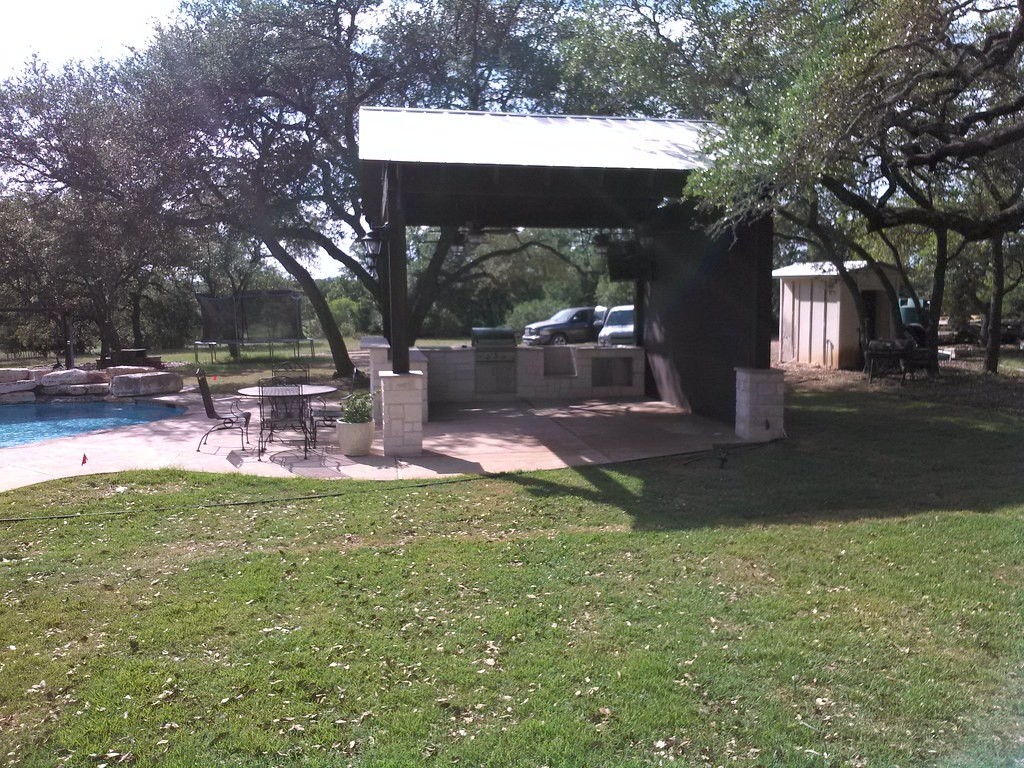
[257,362,317,461]
[311,367,356,449]
[195,368,252,452]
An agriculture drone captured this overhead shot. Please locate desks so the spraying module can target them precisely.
[238,385,337,452]
[193,337,315,363]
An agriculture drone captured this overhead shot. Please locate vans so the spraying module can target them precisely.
[597,304,635,346]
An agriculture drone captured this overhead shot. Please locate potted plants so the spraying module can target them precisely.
[336,389,383,456]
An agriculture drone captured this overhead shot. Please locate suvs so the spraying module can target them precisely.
[521,306,607,346]
